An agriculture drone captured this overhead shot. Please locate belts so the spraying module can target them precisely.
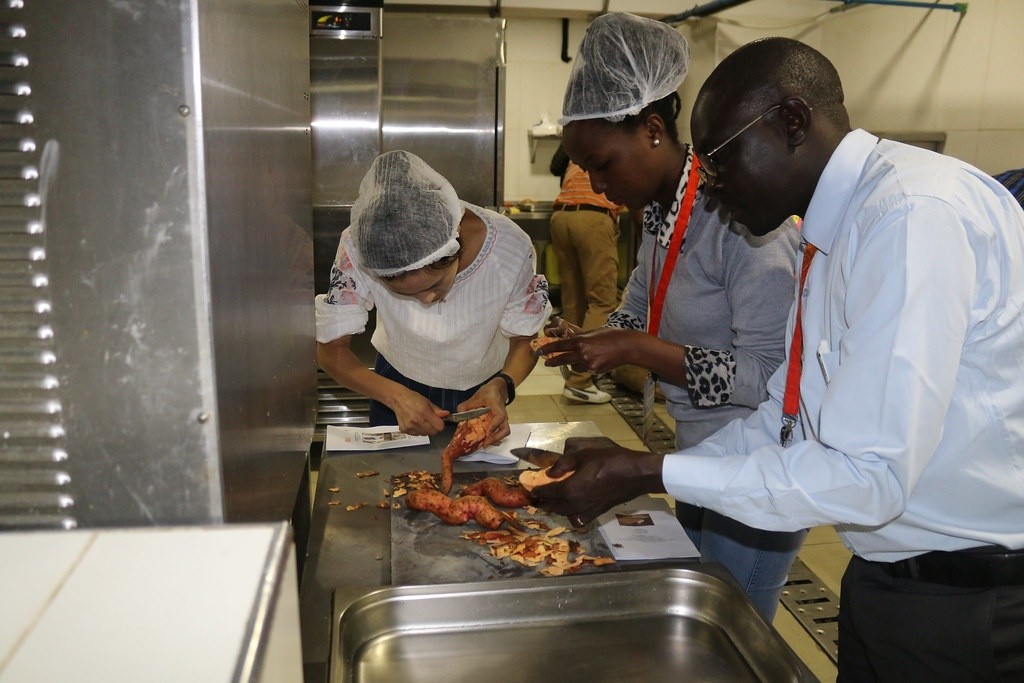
[555,204,616,221]
[876,552,1024,585]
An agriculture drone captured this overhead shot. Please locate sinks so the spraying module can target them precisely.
[499,203,553,243]
[337,567,802,683]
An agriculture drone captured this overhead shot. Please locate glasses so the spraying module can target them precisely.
[695,102,813,184]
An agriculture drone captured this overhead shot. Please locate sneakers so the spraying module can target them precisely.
[559,363,571,383]
[562,383,612,405]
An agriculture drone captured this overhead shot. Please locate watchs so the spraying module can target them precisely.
[498,372,516,405]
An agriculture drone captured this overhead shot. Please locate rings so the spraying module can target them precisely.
[576,517,585,526]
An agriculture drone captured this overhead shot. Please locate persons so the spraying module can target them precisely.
[537,12,812,625]
[549,144,646,403]
[316,151,553,451]
[530,37,1024,683]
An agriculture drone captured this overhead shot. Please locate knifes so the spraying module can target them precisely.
[441,407,492,423]
[510,447,563,468]
